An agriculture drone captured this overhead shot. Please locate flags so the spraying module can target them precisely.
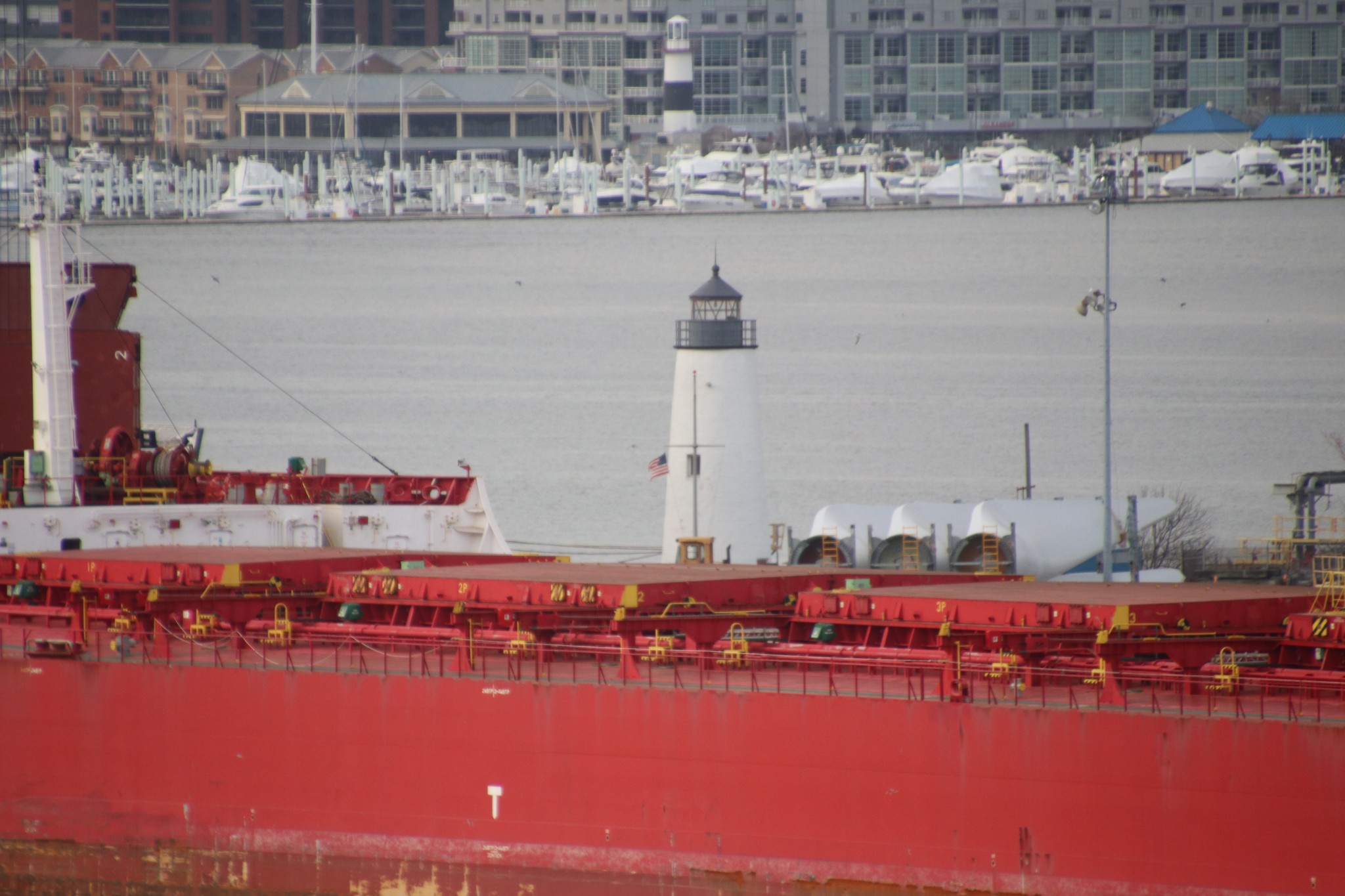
[648,453,669,481]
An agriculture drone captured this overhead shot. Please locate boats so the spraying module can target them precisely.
[0,134,1319,222]
[0,239,1345,896]
